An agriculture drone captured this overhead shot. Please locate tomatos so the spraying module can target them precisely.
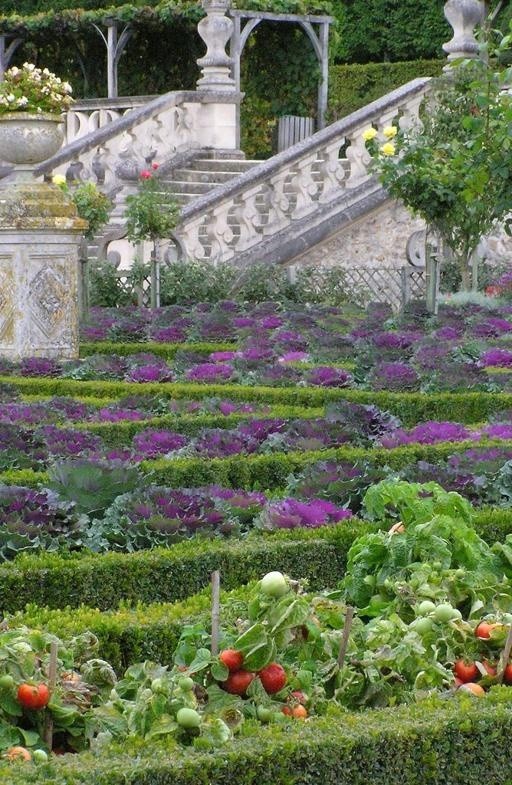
[0,570,512,765]
